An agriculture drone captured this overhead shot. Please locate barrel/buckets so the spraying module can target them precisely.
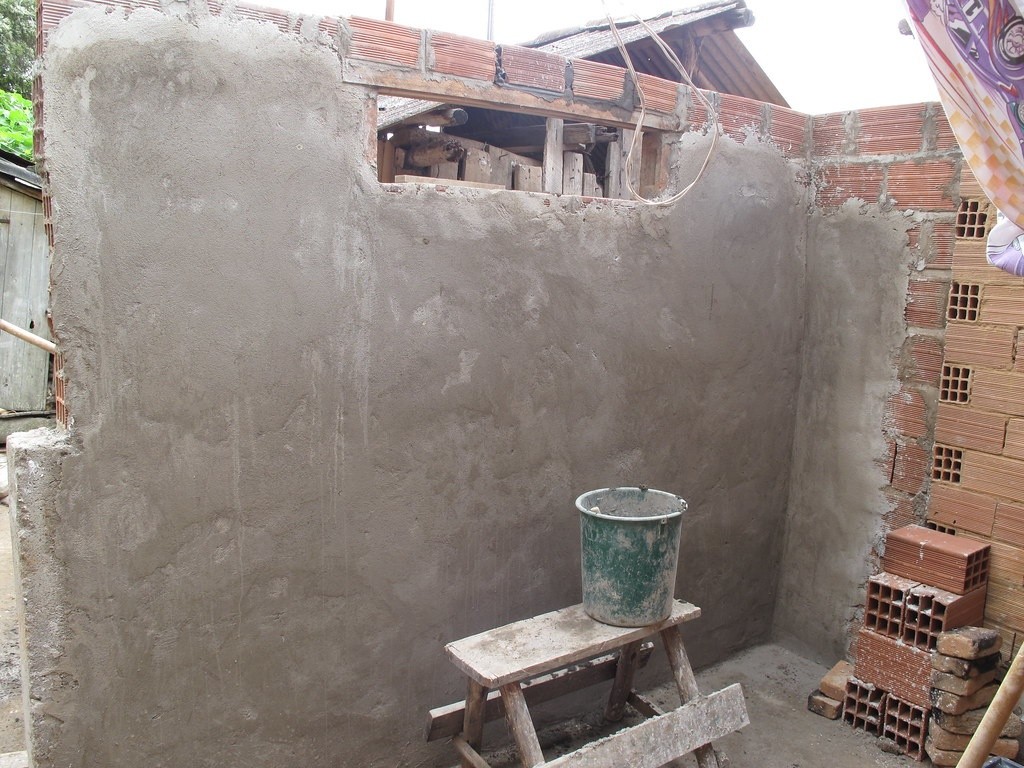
[575,487,688,626]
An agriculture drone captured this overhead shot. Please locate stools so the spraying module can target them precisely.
[423,598,751,768]
